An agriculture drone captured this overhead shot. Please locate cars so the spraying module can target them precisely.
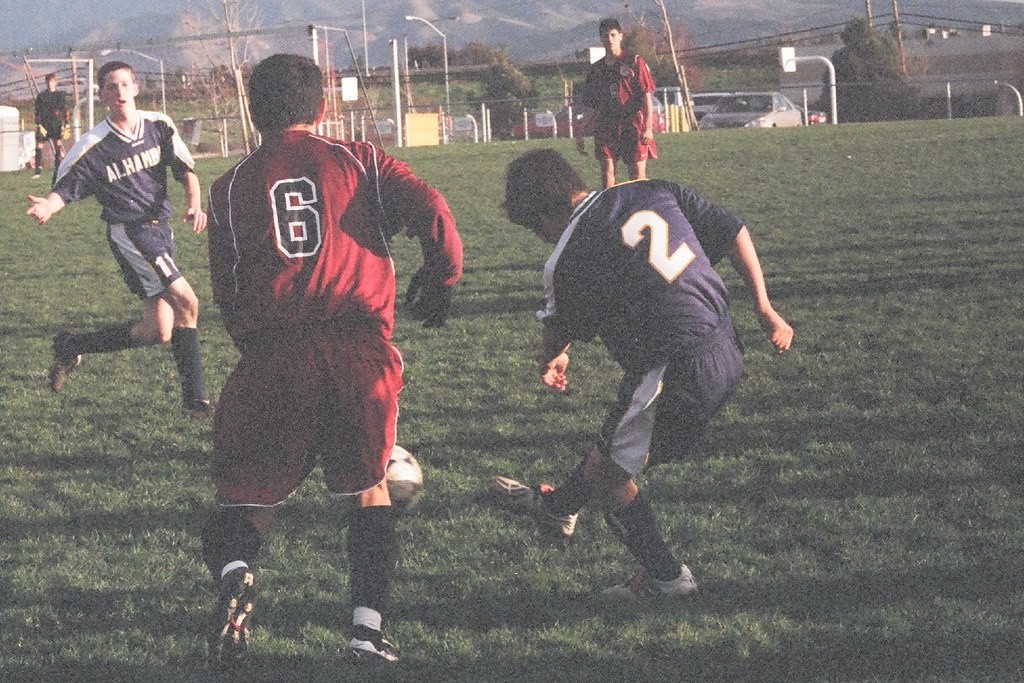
[697,91,802,130]
[508,97,665,138]
[792,103,828,128]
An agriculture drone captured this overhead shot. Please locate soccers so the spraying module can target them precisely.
[383,443,423,516]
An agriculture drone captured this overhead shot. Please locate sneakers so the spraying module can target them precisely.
[602,564,696,599]
[349,624,400,663]
[495,474,579,536]
[203,566,257,663]
[183,399,216,417]
[50,333,83,393]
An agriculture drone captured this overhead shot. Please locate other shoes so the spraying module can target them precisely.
[30,174,42,179]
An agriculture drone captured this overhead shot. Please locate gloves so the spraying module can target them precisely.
[39,126,47,137]
[407,262,453,328]
[62,124,71,139]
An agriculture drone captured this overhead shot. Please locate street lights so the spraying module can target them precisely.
[406,16,450,116]
[99,48,176,120]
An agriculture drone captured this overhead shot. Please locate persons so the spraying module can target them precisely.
[488,146,794,600]
[25,60,217,413]
[574,17,658,190]
[31,73,70,178]
[199,51,463,670]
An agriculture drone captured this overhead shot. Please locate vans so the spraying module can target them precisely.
[691,92,731,121]
[655,86,694,109]
